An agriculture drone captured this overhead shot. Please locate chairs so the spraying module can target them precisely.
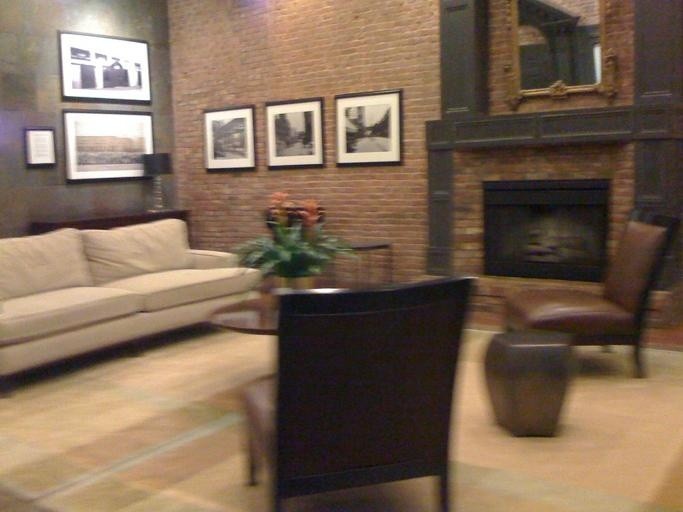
[242,277,477,512]
[499,207,679,378]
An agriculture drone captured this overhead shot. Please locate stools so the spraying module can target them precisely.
[484,332,573,436]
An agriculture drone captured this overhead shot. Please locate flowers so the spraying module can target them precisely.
[233,192,356,274]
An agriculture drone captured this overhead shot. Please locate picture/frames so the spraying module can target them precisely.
[262,96,326,171]
[22,124,59,168]
[334,89,403,167]
[63,108,157,184]
[58,30,151,105]
[201,104,256,172]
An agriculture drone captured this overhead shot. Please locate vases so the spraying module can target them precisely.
[277,272,315,291]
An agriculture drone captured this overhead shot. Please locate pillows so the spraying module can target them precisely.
[1,227,92,295]
[80,216,191,279]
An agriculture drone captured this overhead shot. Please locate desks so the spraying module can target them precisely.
[31,210,191,235]
[210,282,373,336]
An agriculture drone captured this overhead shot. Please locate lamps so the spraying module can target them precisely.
[143,152,175,210]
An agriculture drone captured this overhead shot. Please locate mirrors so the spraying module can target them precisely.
[501,1,621,110]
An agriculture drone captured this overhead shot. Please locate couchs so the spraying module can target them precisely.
[0,219,262,379]
